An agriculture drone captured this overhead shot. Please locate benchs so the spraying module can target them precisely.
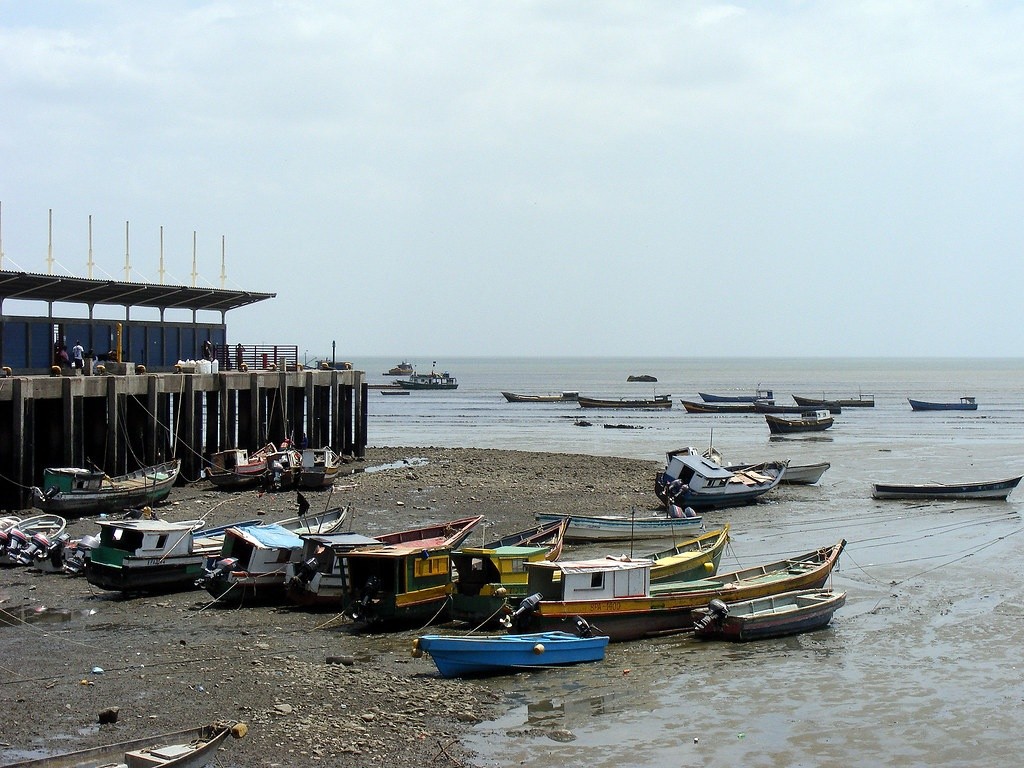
[104,361,137,375]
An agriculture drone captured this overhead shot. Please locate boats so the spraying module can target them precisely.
[765,406,835,434]
[0,415,847,635]
[390,361,459,391]
[501,390,580,403]
[576,384,672,408]
[382,359,414,375]
[753,401,842,415]
[698,382,774,403]
[871,473,1024,502]
[380,391,410,395]
[679,399,757,413]
[31,457,182,518]
[411,627,610,681]
[907,396,978,412]
[690,587,848,644]
[792,386,875,407]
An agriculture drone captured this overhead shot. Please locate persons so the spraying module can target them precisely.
[302,433,308,450]
[60,345,68,362]
[203,340,213,361]
[236,343,246,370]
[73,340,84,369]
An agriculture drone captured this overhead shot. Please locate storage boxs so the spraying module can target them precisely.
[302,448,332,468]
[224,449,248,467]
